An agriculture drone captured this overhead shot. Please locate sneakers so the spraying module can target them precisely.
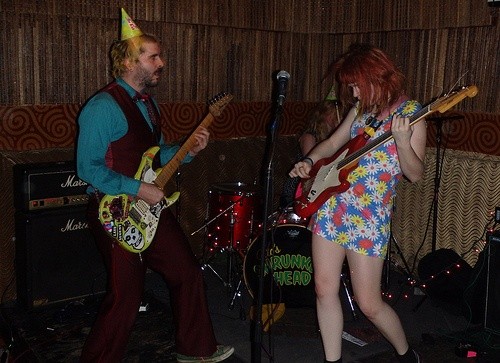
[176,345,234,363]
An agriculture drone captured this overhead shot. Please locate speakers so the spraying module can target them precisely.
[483,234,500,336]
[14,207,112,306]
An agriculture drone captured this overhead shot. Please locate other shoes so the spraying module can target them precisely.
[411,349,420,363]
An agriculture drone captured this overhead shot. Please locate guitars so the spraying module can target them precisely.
[294,84,479,218]
[98,90,235,254]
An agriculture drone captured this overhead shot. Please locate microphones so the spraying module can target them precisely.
[277,70,291,106]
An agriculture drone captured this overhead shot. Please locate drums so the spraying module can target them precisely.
[203,182,262,254]
[243,207,316,310]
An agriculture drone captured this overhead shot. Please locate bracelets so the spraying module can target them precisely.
[303,158,314,165]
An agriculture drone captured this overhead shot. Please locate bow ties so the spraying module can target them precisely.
[133,91,150,102]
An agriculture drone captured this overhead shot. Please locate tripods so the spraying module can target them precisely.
[190,192,250,296]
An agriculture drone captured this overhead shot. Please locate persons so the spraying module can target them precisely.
[289,44,428,363]
[76,7,235,363]
[283,82,346,203]
